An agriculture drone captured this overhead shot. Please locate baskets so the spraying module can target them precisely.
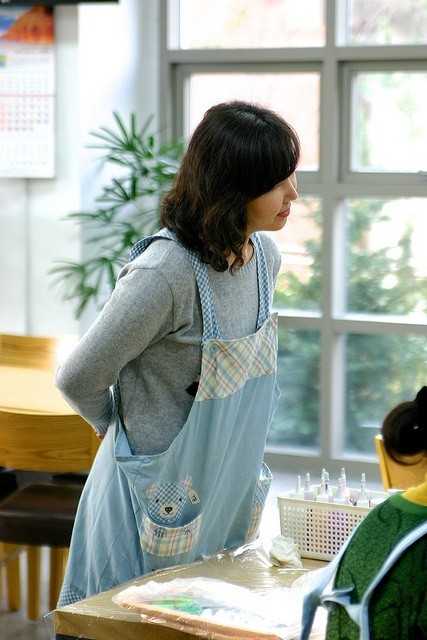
[277,485,391,561]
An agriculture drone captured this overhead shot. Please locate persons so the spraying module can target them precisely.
[54,101,299,640]
[324,385,427,640]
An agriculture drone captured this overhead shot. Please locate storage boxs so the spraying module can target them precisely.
[277,486,375,562]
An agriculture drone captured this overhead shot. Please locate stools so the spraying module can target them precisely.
[0,483,82,622]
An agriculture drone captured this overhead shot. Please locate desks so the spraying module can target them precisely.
[53,546,329,640]
[0,333,100,475]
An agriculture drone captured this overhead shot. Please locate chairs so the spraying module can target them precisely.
[375,434,427,492]
